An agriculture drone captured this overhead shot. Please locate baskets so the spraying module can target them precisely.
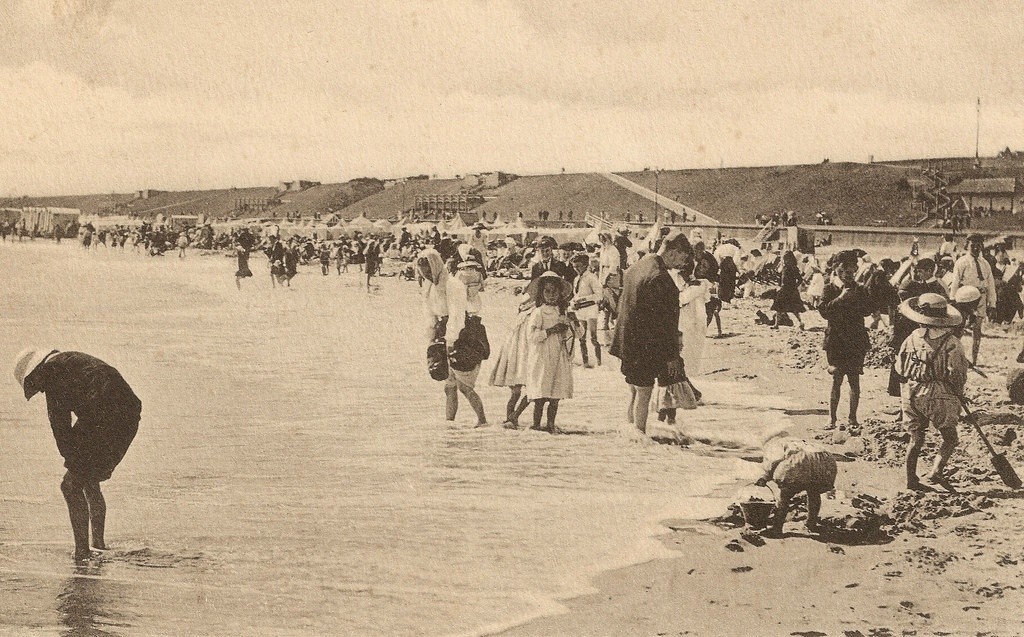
[738,483,777,525]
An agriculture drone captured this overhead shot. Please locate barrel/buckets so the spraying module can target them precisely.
[739,483,777,530]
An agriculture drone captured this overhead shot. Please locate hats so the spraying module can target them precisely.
[15,346,61,400]
[900,294,964,326]
[526,270,574,301]
[968,233,984,242]
[457,255,478,267]
[598,233,613,244]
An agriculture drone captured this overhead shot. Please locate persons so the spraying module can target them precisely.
[528,272,586,435]
[888,280,922,396]
[818,251,872,429]
[415,248,487,425]
[13,346,142,557]
[652,330,704,423]
[571,254,603,368]
[0,210,1023,340]
[609,232,691,432]
[755,430,837,537]
[950,233,997,365]
[892,292,965,488]
[489,307,535,428]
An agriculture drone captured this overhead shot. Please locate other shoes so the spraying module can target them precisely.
[931,471,951,484]
[762,530,781,539]
[908,475,920,487]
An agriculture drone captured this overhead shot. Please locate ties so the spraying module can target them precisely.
[971,254,984,280]
